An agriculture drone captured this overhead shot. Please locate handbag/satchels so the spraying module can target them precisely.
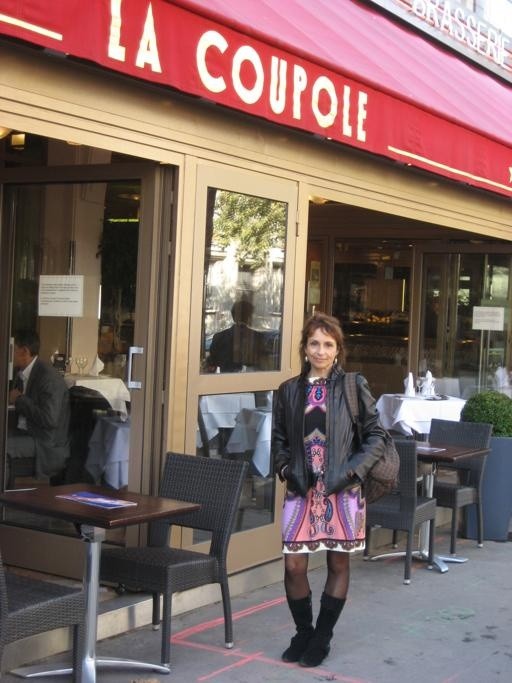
[343,369,402,505]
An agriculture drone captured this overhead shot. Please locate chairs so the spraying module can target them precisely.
[102,451,250,668]
[417,418,495,548]
[362,439,439,584]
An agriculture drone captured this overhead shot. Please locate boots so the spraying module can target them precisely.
[282,591,315,664]
[300,591,347,668]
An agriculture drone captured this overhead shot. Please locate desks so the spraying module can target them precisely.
[376,393,470,436]
[1,483,202,676]
[407,441,493,573]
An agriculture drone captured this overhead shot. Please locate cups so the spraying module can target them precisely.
[65,358,72,375]
[77,356,87,377]
[417,376,424,397]
[430,378,437,398]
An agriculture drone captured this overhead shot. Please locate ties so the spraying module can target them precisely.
[8,376,23,429]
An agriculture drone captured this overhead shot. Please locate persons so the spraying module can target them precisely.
[7,323,73,478]
[270,313,387,667]
[209,301,264,371]
